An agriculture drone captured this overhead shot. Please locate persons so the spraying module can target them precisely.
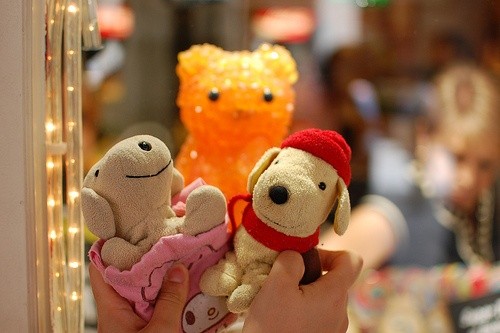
[309,58,500,278]
[88,249,364,333]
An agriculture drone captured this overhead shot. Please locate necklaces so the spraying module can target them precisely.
[416,179,493,267]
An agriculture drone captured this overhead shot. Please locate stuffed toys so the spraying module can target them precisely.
[82,133,247,332]
[198,128,355,315]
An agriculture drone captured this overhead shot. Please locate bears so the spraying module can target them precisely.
[173,42,300,232]
